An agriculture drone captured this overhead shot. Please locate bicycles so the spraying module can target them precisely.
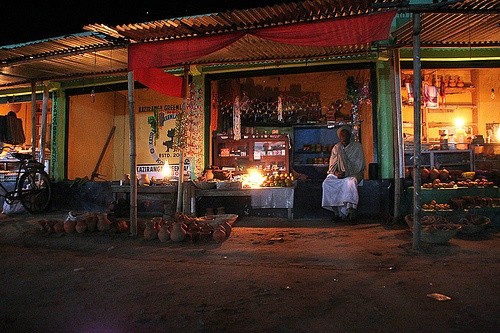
[0,145,52,215]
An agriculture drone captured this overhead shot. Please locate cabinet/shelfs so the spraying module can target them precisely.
[35,100,52,149]
[401,69,500,173]
[214,121,354,176]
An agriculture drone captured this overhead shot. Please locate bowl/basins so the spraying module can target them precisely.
[193,181,217,190]
[198,214,238,226]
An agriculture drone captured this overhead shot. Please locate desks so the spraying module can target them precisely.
[191,185,295,221]
[404,177,500,227]
[111,184,179,217]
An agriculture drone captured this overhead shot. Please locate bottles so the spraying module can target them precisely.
[39,212,127,234]
[187,220,232,244]
[143,214,186,243]
[440,134,455,150]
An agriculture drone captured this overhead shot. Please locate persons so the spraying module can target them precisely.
[321,124,364,224]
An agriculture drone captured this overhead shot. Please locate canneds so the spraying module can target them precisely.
[307,144,328,164]
